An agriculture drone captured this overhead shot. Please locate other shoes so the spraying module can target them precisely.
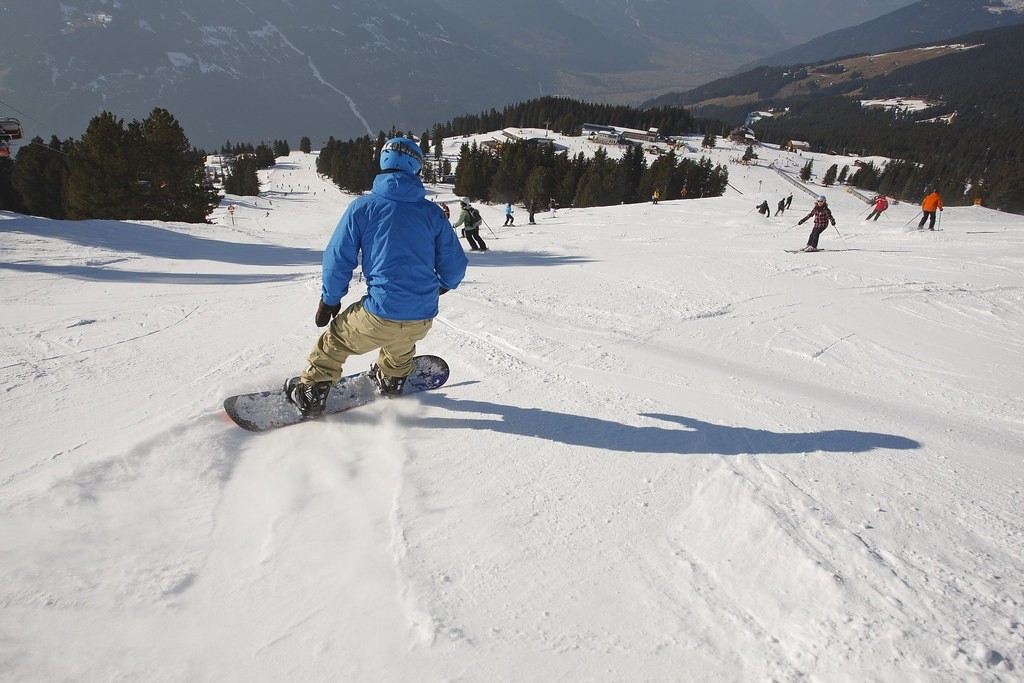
[369,358,406,396]
[285,372,331,414]
[802,244,817,252]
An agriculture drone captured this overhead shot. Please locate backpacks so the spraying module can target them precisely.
[468,209,483,230]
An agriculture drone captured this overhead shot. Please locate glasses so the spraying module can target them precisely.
[818,201,824,203]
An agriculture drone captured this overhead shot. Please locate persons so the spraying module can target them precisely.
[785,191,793,209]
[681,185,687,198]
[528,198,537,225]
[284,137,468,419]
[918,189,943,230]
[866,194,888,221]
[453,197,487,251]
[504,203,514,226]
[775,198,785,217]
[548,197,556,217]
[757,200,770,218]
[798,196,835,251]
[653,191,658,204]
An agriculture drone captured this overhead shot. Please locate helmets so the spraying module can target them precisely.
[934,188,939,193]
[461,196,471,206]
[818,196,826,203]
[379,135,423,180]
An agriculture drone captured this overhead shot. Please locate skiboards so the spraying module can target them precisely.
[467,247,491,252]
[905,228,944,232]
[783,247,825,253]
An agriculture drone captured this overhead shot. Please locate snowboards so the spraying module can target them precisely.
[223,353,449,433]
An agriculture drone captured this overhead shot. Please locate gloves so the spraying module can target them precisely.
[439,285,448,295]
[314,298,342,327]
[798,219,805,225]
[831,221,835,225]
